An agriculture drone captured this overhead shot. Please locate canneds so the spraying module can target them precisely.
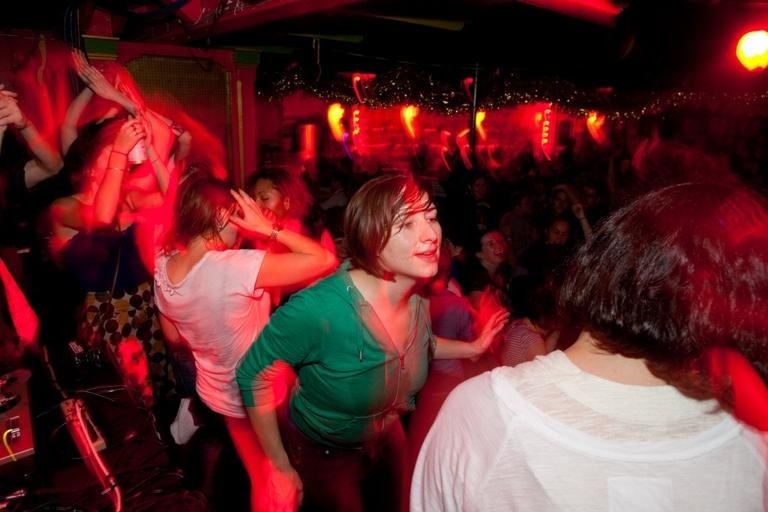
[128,138,146,165]
